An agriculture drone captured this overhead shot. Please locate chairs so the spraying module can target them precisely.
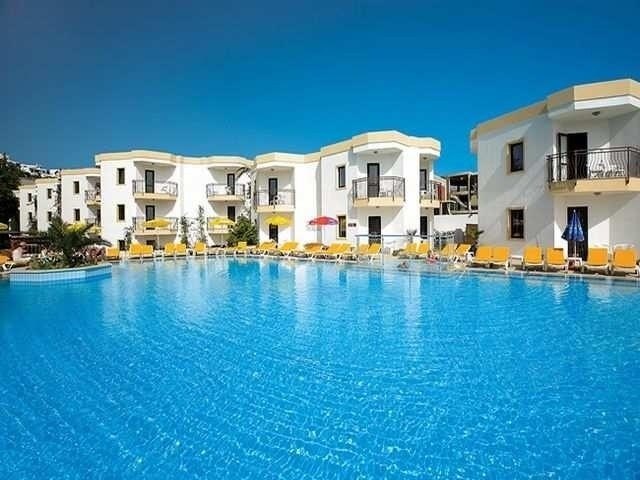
[397,242,640,278]
[588,161,625,178]
[100,239,385,266]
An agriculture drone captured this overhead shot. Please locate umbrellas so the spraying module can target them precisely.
[210,216,235,244]
[142,218,172,248]
[307,215,339,244]
[263,216,291,242]
[66,222,104,234]
[560,209,586,257]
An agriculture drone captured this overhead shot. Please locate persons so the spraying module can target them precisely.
[11,241,106,265]
